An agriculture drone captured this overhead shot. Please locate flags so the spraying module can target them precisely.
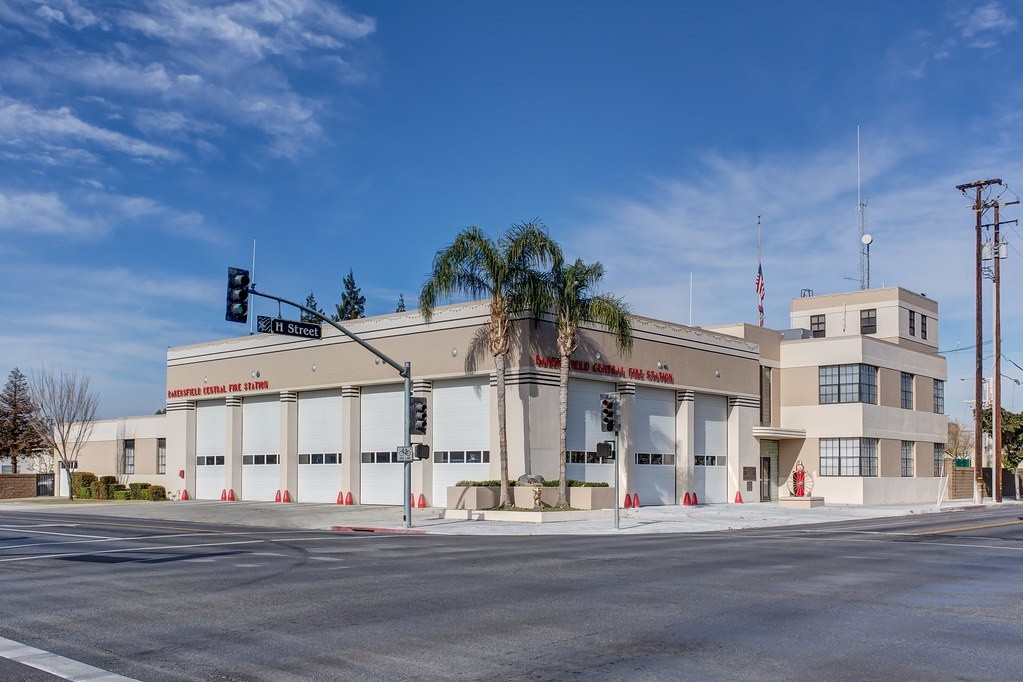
[756,263,765,301]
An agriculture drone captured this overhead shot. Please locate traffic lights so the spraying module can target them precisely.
[408,396,427,435]
[225,267,250,323]
[600,392,622,432]
